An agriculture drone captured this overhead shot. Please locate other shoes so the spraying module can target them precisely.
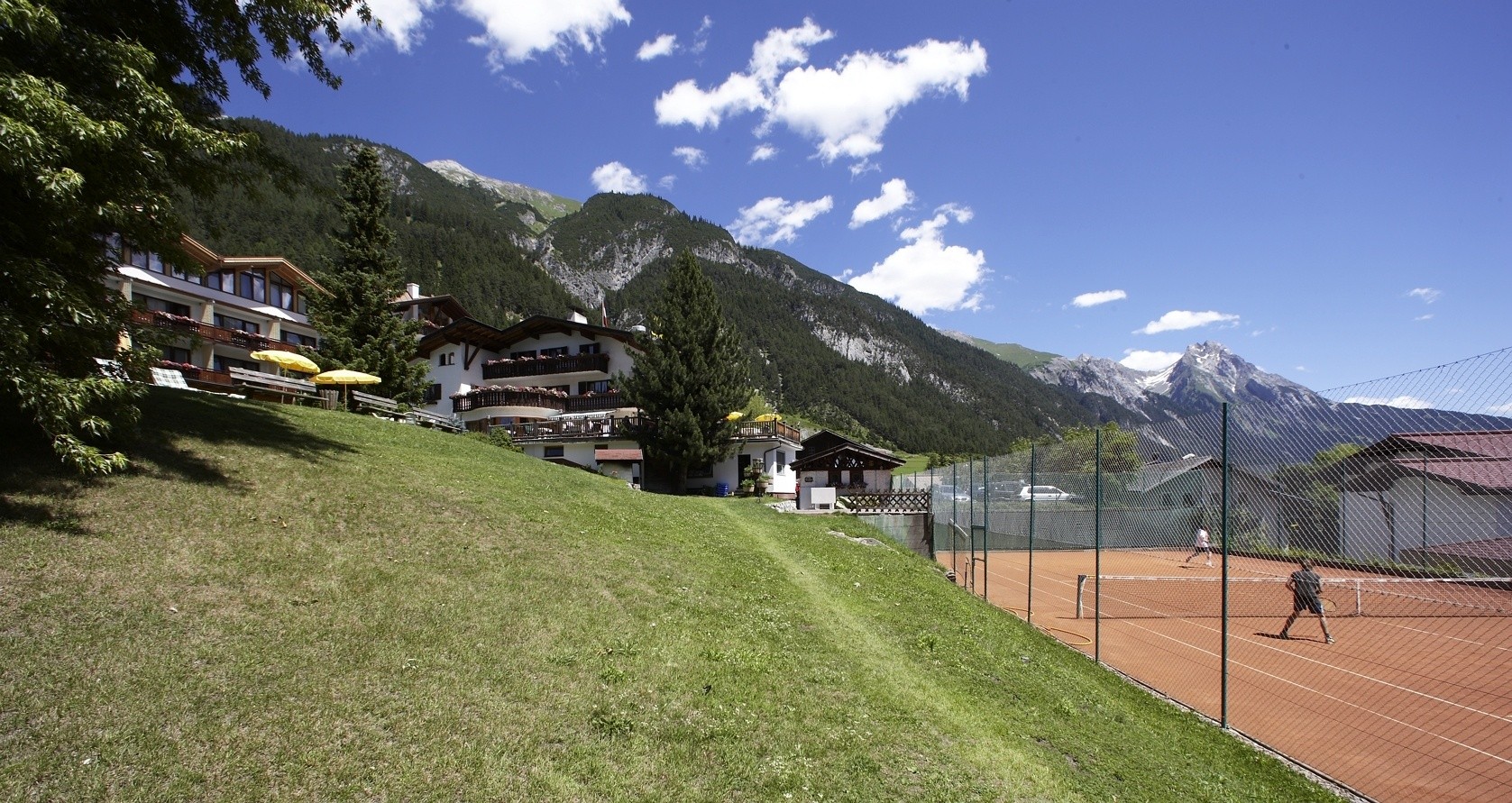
[1279,630,1290,640]
[1205,562,1215,569]
[1325,636,1336,643]
[1182,562,1190,568]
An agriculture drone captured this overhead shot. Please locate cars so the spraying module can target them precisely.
[936,485,970,502]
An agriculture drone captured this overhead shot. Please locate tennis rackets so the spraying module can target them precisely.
[1294,590,1337,612]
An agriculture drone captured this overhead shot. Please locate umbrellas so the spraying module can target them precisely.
[755,414,782,421]
[309,369,381,411]
[250,350,321,404]
[726,412,745,420]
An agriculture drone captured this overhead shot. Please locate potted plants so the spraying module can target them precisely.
[743,465,753,477]
[740,478,756,492]
[762,475,773,484]
[756,478,768,488]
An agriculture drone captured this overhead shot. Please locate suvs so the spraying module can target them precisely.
[967,482,1076,507]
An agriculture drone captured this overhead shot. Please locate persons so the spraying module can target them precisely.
[1186,524,1214,568]
[1280,560,1334,644]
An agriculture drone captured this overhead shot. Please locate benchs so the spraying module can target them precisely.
[412,407,461,433]
[352,390,411,421]
[228,366,329,406]
